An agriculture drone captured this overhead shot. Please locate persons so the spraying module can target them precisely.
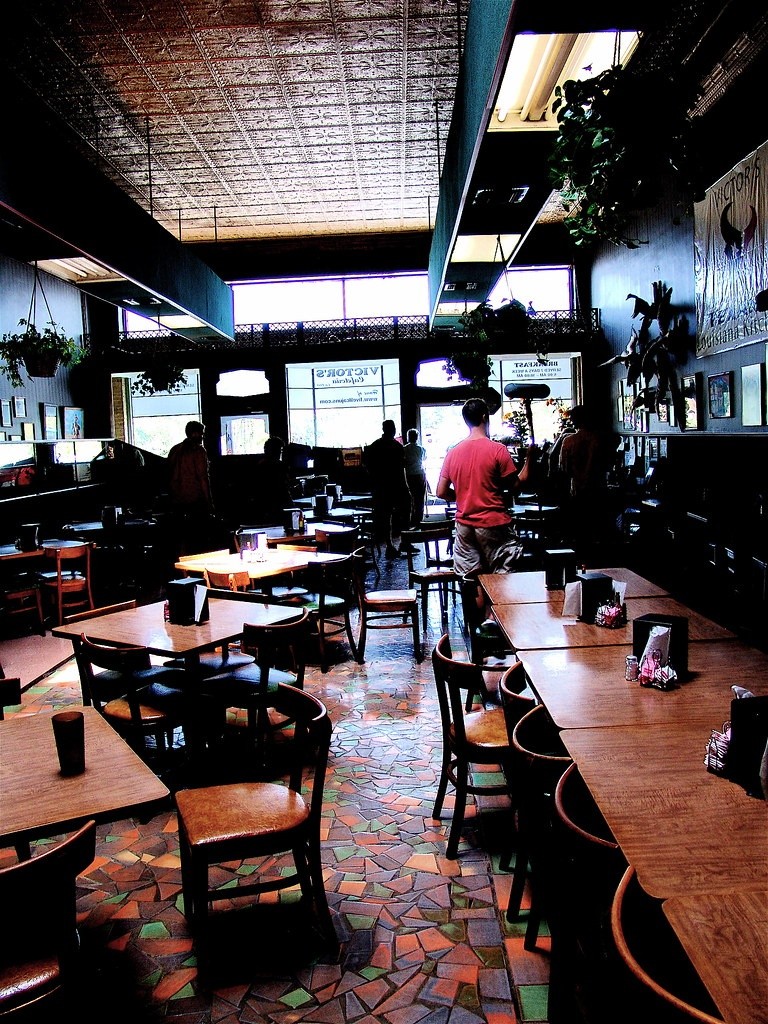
[402,429,426,522]
[168,422,209,504]
[435,399,544,621]
[367,420,421,557]
[558,405,606,497]
[254,436,303,522]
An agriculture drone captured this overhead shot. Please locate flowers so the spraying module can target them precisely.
[501,398,528,445]
[547,396,570,431]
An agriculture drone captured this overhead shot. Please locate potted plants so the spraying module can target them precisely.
[546,64,708,248]
[132,361,189,396]
[459,299,539,355]
[0,319,86,388]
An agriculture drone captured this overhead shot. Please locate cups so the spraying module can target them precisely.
[52,712,86,776]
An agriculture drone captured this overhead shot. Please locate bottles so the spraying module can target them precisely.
[624,655,639,680]
[242,542,254,563]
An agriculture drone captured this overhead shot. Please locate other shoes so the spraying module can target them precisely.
[385,546,401,557]
[399,543,420,552]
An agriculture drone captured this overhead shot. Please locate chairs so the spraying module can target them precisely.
[0,520,735,1024]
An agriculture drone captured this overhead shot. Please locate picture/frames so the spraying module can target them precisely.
[14,396,27,418]
[617,379,648,433]
[43,403,58,439]
[669,404,676,427]
[656,398,668,422]
[679,372,701,431]
[9,435,22,441]
[740,363,762,427]
[0,431,6,441]
[707,371,733,419]
[22,422,35,440]
[64,407,85,439]
[0,399,13,427]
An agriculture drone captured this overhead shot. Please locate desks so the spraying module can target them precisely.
[300,508,371,520]
[491,597,737,652]
[515,640,768,728]
[0,706,171,864]
[52,598,306,747]
[175,549,349,590]
[230,523,354,548]
[292,496,371,502]
[477,566,671,605]
[556,722,768,897]
[0,537,85,585]
[663,887,768,1024]
